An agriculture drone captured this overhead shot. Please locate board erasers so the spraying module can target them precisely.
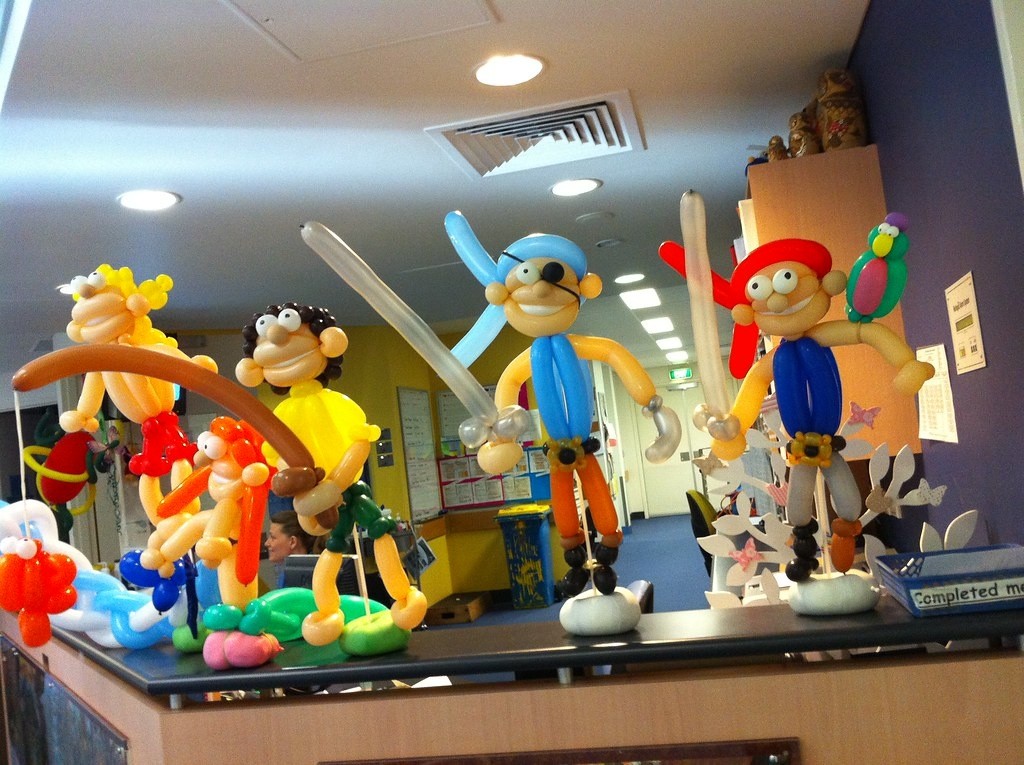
[438,508,448,516]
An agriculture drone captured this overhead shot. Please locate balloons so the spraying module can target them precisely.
[0,188,936,668]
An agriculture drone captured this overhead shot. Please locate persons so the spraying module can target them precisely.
[265,511,317,590]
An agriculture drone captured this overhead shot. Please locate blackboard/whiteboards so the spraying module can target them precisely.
[396,385,446,525]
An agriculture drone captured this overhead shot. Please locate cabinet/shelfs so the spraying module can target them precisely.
[747,142,923,464]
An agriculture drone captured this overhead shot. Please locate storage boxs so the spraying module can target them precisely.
[426,591,493,627]
[876,543,1024,618]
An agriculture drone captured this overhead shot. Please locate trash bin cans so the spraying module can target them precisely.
[492,505,555,609]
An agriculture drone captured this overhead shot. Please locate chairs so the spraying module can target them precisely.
[687,489,718,578]
